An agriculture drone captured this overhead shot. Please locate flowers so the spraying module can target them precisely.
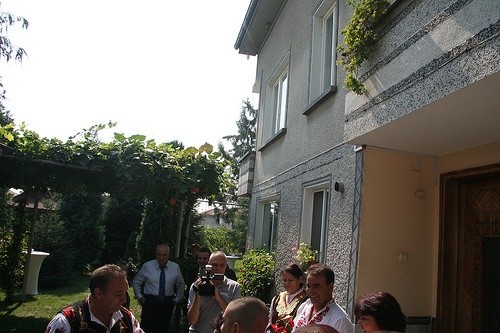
[240,244,304,286]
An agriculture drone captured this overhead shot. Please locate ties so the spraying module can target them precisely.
[158,266,168,303]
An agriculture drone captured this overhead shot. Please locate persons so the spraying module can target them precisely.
[220,295,269,333]
[186,244,201,264]
[44,264,146,333]
[132,244,185,333]
[291,263,353,333]
[265,263,309,333]
[210,311,224,333]
[182,247,211,319]
[292,324,339,333]
[187,251,241,333]
[355,290,408,333]
